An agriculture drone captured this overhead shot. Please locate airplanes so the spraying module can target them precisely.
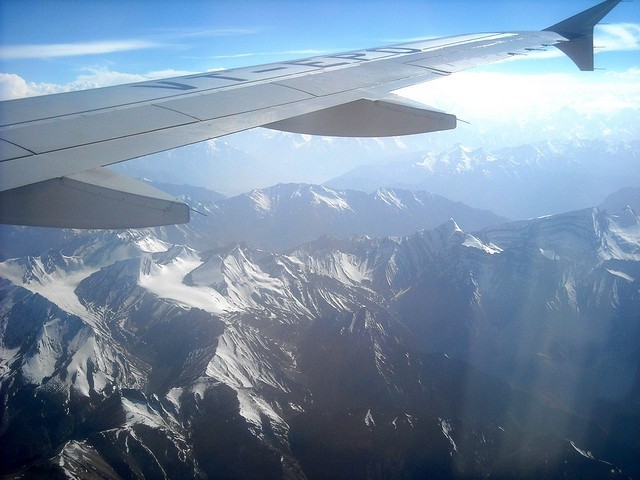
[0,0,620,229]
